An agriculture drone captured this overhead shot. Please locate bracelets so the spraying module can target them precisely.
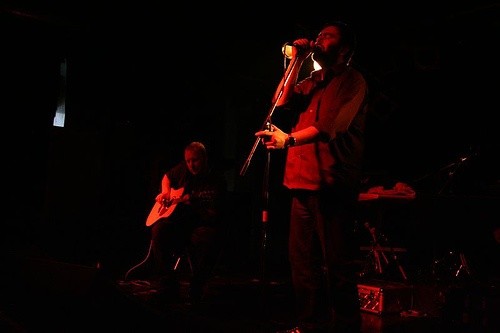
[288,133,295,147]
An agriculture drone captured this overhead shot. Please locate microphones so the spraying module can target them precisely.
[287,41,318,51]
[440,151,479,172]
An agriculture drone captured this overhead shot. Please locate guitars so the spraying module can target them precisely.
[144,184,218,227]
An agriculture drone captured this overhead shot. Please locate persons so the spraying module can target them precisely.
[254,23,369,333]
[124,141,240,306]
